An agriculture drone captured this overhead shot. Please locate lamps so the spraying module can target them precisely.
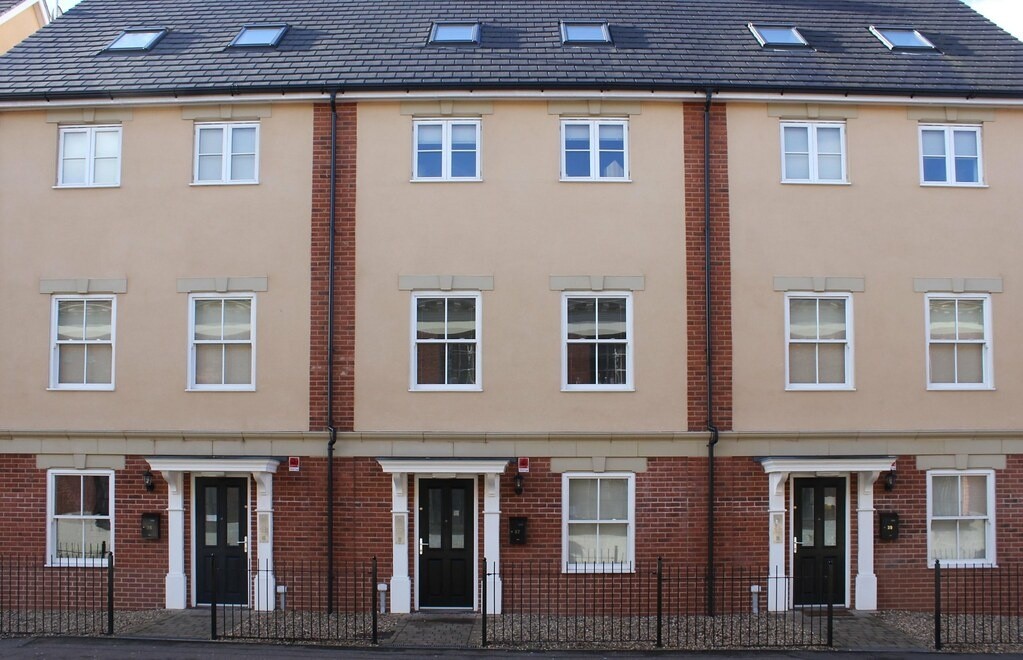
[142,468,153,489]
[886,468,897,492]
[513,473,523,495]
